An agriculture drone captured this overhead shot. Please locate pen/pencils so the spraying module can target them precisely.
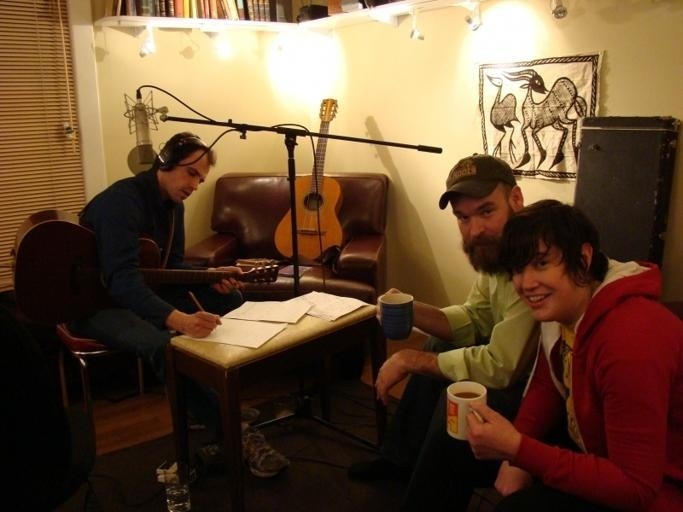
[189,291,205,311]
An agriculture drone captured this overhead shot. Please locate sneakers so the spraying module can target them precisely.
[348,450,397,480]
[241,430,290,484]
[240,407,259,420]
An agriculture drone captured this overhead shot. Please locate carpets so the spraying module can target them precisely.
[56,380,504,512]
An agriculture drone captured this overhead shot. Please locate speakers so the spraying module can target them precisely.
[574,119,678,266]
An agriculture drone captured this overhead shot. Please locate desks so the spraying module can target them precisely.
[167,292,387,512]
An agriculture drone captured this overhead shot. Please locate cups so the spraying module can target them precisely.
[446,381,489,441]
[377,293,413,340]
[165,483,191,512]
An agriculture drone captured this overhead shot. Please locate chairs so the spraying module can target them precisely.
[183,172,387,392]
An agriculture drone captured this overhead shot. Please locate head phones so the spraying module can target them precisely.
[155,136,211,170]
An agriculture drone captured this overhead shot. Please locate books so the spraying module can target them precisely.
[101,0,293,24]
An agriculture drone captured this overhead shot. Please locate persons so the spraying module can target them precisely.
[462,194,682,511]
[61,130,294,483]
[348,149,544,510]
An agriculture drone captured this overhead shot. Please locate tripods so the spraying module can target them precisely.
[123,91,442,454]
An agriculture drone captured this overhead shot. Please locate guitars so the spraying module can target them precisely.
[10,210,278,322]
[275,99,343,267]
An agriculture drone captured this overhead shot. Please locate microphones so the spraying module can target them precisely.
[134,103,155,164]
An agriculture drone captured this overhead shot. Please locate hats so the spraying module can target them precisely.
[438,152,516,210]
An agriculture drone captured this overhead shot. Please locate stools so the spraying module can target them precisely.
[57,321,169,429]
